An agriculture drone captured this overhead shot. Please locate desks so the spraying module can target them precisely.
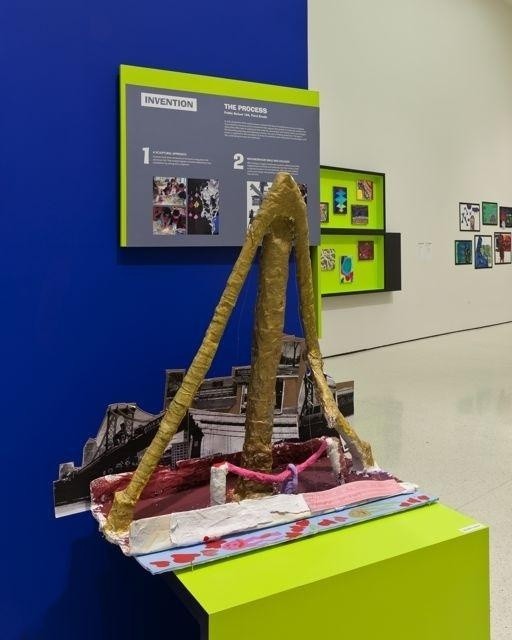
[149,492,491,640]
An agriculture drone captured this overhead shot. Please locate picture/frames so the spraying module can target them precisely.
[454,200,512,268]
